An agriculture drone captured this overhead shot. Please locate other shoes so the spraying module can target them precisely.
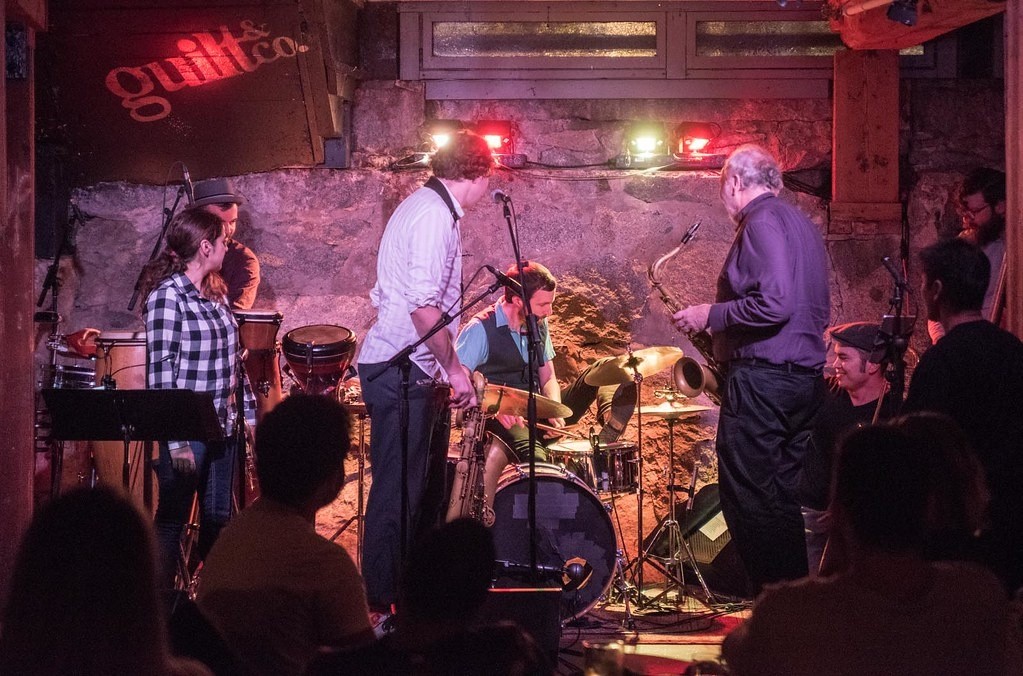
[599,380,637,443]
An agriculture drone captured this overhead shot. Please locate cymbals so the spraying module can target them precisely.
[583,346,684,386]
[449,383,573,419]
[631,403,714,416]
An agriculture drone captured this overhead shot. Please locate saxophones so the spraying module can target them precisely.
[648,217,726,407]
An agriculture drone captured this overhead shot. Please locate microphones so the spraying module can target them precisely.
[685,466,697,514]
[881,256,914,294]
[590,429,602,477]
[486,267,523,296]
[67,191,86,227]
[182,163,196,205]
[490,189,509,204]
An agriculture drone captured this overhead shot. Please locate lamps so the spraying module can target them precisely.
[418,118,464,153]
[624,119,668,161]
[672,118,722,162]
[478,120,515,157]
[887,0,918,26]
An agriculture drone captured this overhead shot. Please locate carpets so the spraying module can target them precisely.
[559,607,753,645]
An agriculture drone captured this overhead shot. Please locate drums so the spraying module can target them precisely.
[231,308,285,429]
[167,440,198,474]
[483,462,621,625]
[280,324,357,402]
[44,364,96,390]
[92,328,163,525]
[545,439,640,495]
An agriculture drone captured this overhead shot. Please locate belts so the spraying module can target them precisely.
[731,358,810,372]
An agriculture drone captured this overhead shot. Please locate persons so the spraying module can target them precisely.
[721,412,1023,676]
[143,209,256,580]
[358,131,494,615]
[304,517,552,676]
[901,239,1023,589]
[0,484,213,676]
[195,395,376,676]
[184,177,260,312]
[804,320,906,513]
[671,144,827,595]
[927,166,1006,343]
[454,262,638,465]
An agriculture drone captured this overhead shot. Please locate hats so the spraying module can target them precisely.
[184,177,242,210]
[830,322,887,352]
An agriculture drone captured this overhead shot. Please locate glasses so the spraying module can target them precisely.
[959,199,992,220]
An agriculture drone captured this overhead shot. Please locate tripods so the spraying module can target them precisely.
[609,372,715,632]
[327,416,366,571]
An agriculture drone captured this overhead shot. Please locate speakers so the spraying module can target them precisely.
[642,482,752,598]
